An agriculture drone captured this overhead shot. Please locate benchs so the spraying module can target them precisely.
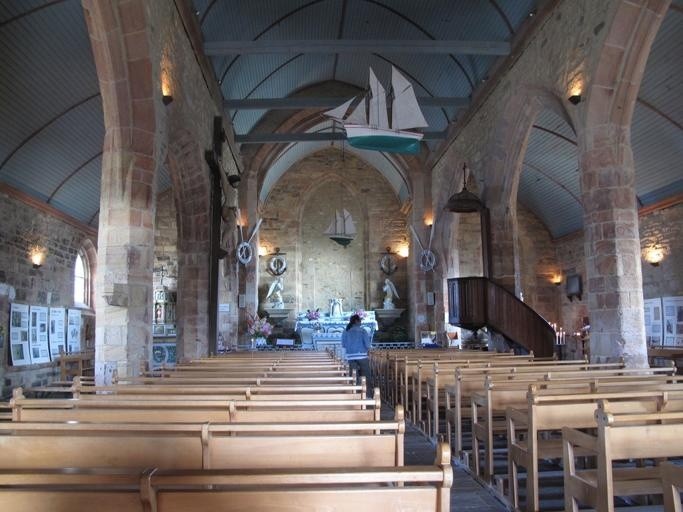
[0,346,683,511]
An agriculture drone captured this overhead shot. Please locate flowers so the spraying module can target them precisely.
[245,312,273,338]
[304,308,319,320]
[351,308,367,319]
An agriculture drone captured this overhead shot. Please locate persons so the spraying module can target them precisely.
[342,315,373,398]
[382,278,392,303]
[273,277,283,302]
[217,157,241,249]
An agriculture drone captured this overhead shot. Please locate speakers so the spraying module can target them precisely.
[239,294,246,308]
[427,292,434,305]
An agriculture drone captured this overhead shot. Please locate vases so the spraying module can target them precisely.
[255,336,267,347]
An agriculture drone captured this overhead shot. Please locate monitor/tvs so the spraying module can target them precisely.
[566,274,582,297]
